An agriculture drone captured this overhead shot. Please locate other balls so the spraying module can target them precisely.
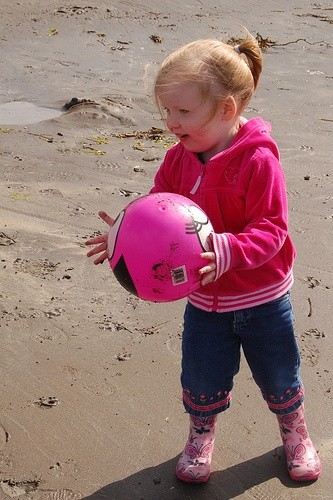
[107,193,215,303]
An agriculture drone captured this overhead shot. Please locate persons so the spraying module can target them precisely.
[85,38,322,480]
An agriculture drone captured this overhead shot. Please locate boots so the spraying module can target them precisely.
[277,402,320,481]
[176,413,218,484]
